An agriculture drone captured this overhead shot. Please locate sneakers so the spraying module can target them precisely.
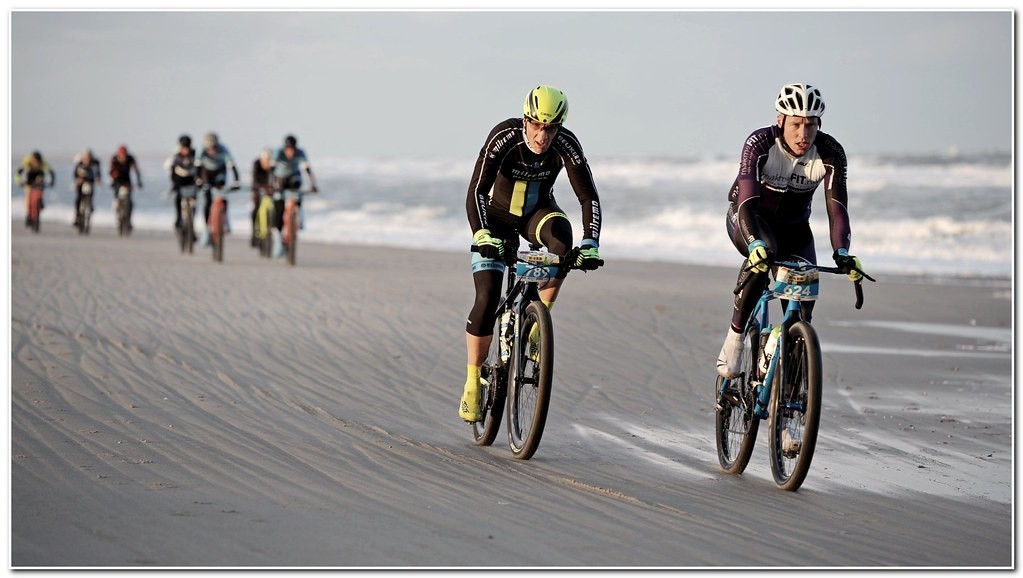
[782,417,804,452]
[717,327,745,379]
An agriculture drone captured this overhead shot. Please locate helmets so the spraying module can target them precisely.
[523,86,569,124]
[774,82,825,119]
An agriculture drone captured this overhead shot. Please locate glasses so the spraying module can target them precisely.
[523,117,561,134]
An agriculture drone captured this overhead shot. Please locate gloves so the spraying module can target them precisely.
[473,229,506,260]
[832,249,864,285]
[573,244,599,272]
[747,240,769,272]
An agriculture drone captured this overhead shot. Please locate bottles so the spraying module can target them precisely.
[756,324,782,380]
[500,308,513,364]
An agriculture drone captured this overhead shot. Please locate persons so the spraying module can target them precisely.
[167,133,319,254]
[715,82,864,453]
[15,151,55,228]
[72,149,102,227]
[108,146,142,232]
[458,85,601,420]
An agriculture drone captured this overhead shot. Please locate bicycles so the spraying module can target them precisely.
[256,195,273,256]
[79,181,91,234]
[272,188,313,265]
[469,245,604,461]
[180,186,196,253]
[715,256,877,491]
[116,185,127,236]
[29,199,39,232]
[199,183,240,261]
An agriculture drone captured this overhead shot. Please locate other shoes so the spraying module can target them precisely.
[457,383,483,422]
[528,332,539,363]
